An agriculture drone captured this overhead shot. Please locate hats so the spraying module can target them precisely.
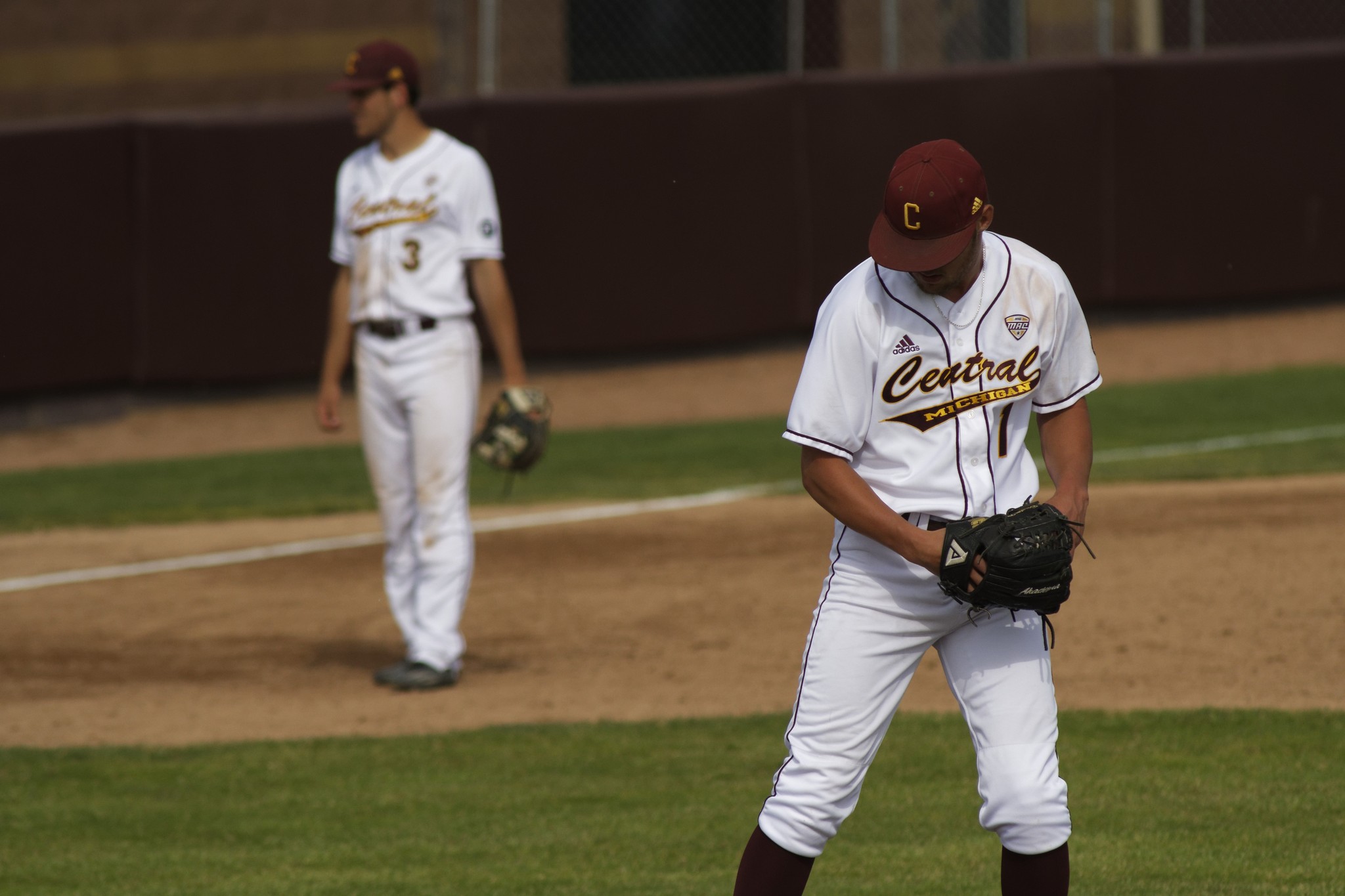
[327,41,422,91]
[869,138,987,273]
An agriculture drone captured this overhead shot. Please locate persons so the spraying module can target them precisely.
[732,137,1103,896]
[311,39,551,691]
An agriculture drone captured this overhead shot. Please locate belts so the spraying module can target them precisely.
[901,511,947,532]
[368,315,435,338]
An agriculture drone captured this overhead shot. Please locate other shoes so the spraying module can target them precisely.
[395,666,462,687]
[378,662,416,684]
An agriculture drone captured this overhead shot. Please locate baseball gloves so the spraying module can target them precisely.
[468,385,552,480]
[938,500,1076,616]
